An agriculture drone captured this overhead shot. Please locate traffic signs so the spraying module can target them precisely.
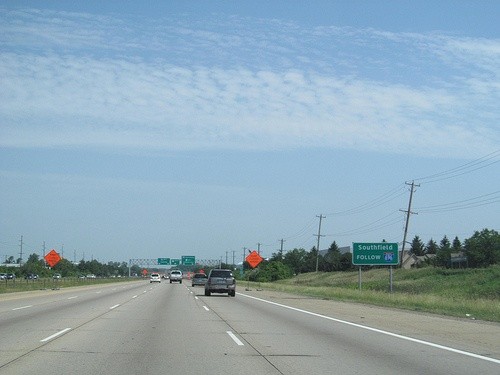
[170,258,180,266]
[181,256,196,266]
[157,257,170,265]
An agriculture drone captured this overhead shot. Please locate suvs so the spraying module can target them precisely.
[168,269,183,284]
[204,268,236,297]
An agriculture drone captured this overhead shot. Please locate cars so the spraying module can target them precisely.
[52,273,62,279]
[0,273,6,280]
[24,274,39,279]
[160,274,170,279]
[86,273,97,279]
[191,273,208,287]
[5,273,15,279]
[149,273,161,283]
[109,273,147,279]
[78,275,86,279]
